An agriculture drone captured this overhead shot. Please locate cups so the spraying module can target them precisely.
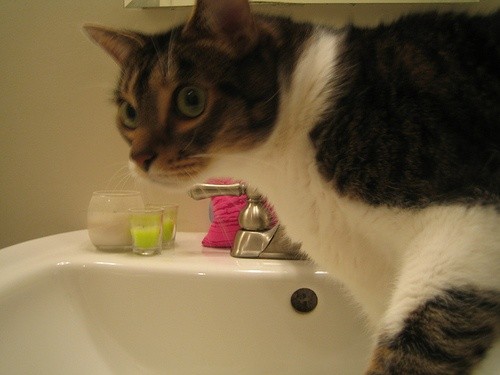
[145,204,178,250]
[87,190,143,252]
[130,207,164,256]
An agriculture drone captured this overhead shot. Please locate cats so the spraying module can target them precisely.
[82,0,500,375]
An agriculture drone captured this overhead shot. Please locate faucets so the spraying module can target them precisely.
[190,182,309,262]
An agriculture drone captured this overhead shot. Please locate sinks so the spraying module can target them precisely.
[0,227,377,375]
